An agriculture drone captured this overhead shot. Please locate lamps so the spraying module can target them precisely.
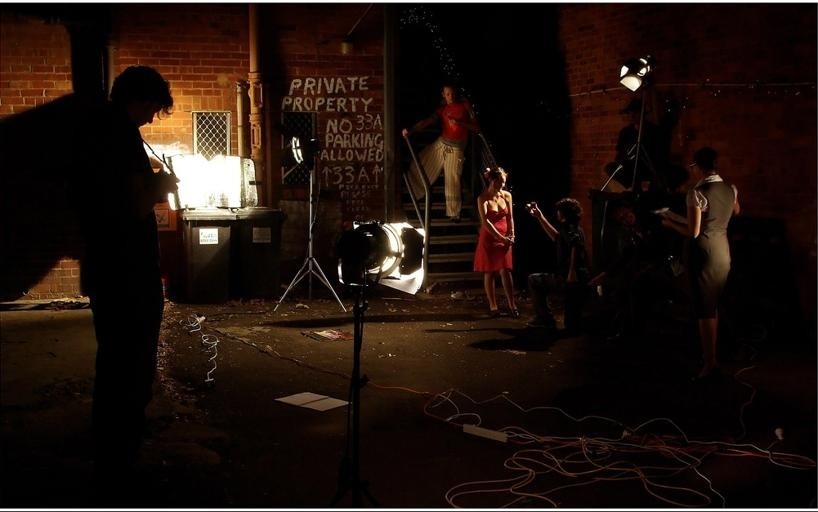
[289,133,322,167]
[334,218,407,289]
[616,55,653,95]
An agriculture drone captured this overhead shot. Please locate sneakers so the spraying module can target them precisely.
[524,316,558,329]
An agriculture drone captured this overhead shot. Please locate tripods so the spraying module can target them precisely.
[601,88,670,193]
[273,170,348,313]
[317,290,390,507]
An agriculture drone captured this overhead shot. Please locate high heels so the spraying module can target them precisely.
[488,305,522,319]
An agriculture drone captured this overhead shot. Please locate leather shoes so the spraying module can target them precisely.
[690,365,720,385]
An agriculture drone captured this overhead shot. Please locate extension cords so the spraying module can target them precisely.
[462,423,508,443]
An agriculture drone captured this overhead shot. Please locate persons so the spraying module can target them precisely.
[78,63,174,443]
[660,145,743,385]
[603,98,689,195]
[400,83,480,223]
[524,197,588,329]
[475,166,523,319]
